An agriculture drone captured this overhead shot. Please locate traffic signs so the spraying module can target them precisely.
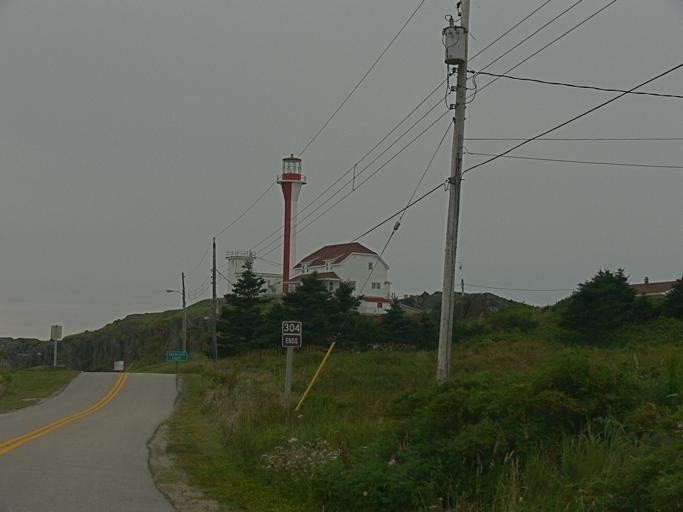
[166,351,186,361]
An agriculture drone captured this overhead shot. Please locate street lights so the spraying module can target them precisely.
[165,289,187,353]
